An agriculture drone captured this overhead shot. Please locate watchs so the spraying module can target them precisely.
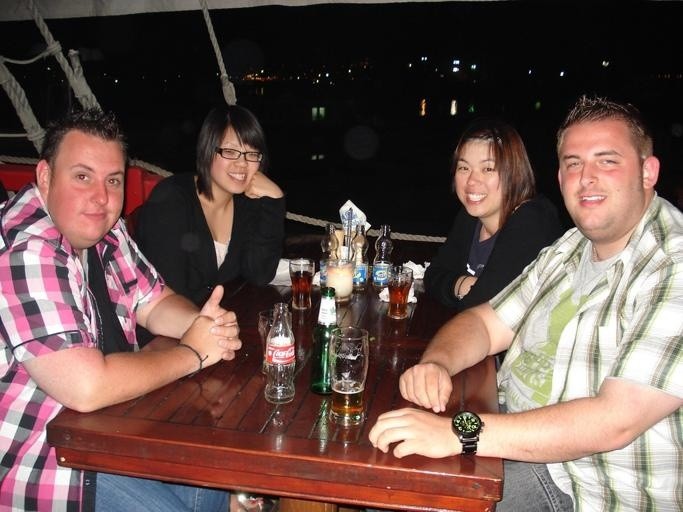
[450,409,483,458]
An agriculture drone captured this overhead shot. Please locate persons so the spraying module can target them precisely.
[124,102,284,308]
[421,124,561,314]
[367,98,683,512]
[0,105,241,512]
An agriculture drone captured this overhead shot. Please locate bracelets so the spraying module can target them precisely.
[174,340,208,381]
[454,274,470,300]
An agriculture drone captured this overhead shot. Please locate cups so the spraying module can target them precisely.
[327,326,369,427]
[386,266,413,320]
[256,311,292,375]
[287,257,315,312]
[326,257,355,302]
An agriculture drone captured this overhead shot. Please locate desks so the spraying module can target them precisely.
[43,282,500,512]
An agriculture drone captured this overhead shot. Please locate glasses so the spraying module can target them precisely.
[216,148,264,162]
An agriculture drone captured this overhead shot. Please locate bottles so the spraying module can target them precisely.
[308,287,341,396]
[347,224,369,291]
[372,225,395,288]
[263,303,295,406]
[318,223,339,291]
